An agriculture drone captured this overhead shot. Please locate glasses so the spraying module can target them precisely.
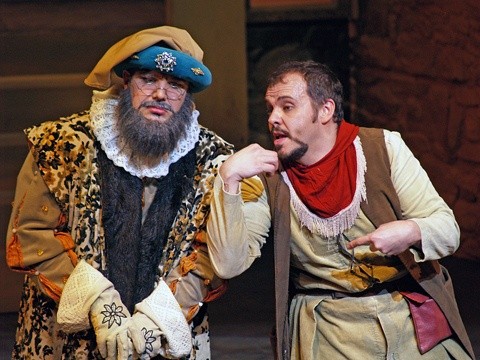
[130,75,186,102]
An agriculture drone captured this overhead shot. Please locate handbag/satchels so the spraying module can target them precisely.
[404,296,452,354]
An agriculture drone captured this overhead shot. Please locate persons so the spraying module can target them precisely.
[206,41,475,360]
[6,26,234,360]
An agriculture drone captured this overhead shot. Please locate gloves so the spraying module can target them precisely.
[129,311,161,360]
[90,289,146,360]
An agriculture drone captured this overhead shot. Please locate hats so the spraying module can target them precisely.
[85,26,213,90]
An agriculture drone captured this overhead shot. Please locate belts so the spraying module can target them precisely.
[296,279,412,299]
